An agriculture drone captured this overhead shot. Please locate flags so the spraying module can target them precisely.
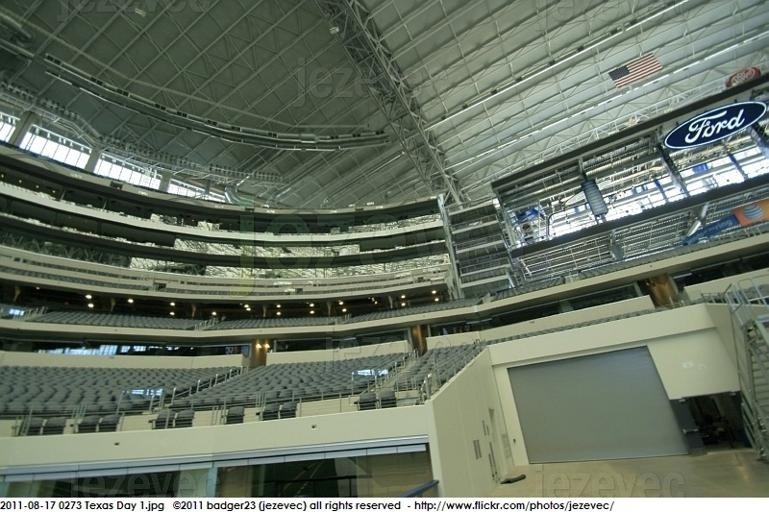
[608,53,663,89]
